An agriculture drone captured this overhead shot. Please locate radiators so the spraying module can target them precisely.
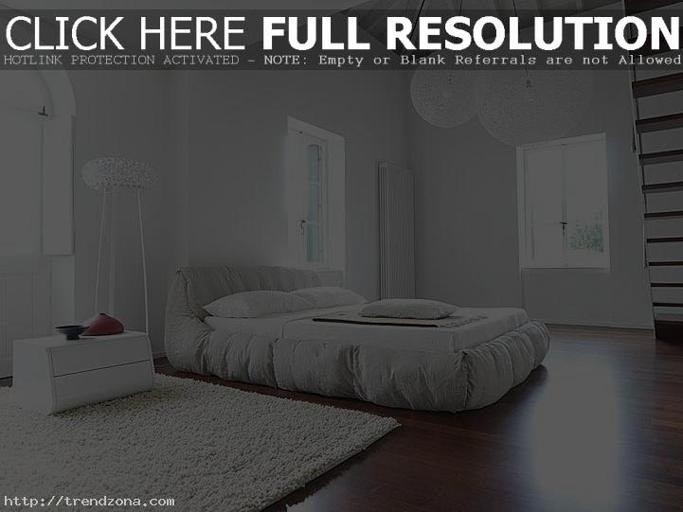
[376,161,415,300]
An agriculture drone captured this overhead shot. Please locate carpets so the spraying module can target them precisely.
[0,370,403,512]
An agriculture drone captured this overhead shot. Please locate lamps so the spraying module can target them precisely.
[408,1,606,153]
[81,156,159,338]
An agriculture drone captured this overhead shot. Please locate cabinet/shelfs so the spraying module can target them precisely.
[8,329,158,416]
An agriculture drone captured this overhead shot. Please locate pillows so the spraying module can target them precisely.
[358,297,461,320]
[287,286,365,312]
[202,291,312,317]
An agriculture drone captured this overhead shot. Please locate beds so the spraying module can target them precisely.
[162,263,554,414]
